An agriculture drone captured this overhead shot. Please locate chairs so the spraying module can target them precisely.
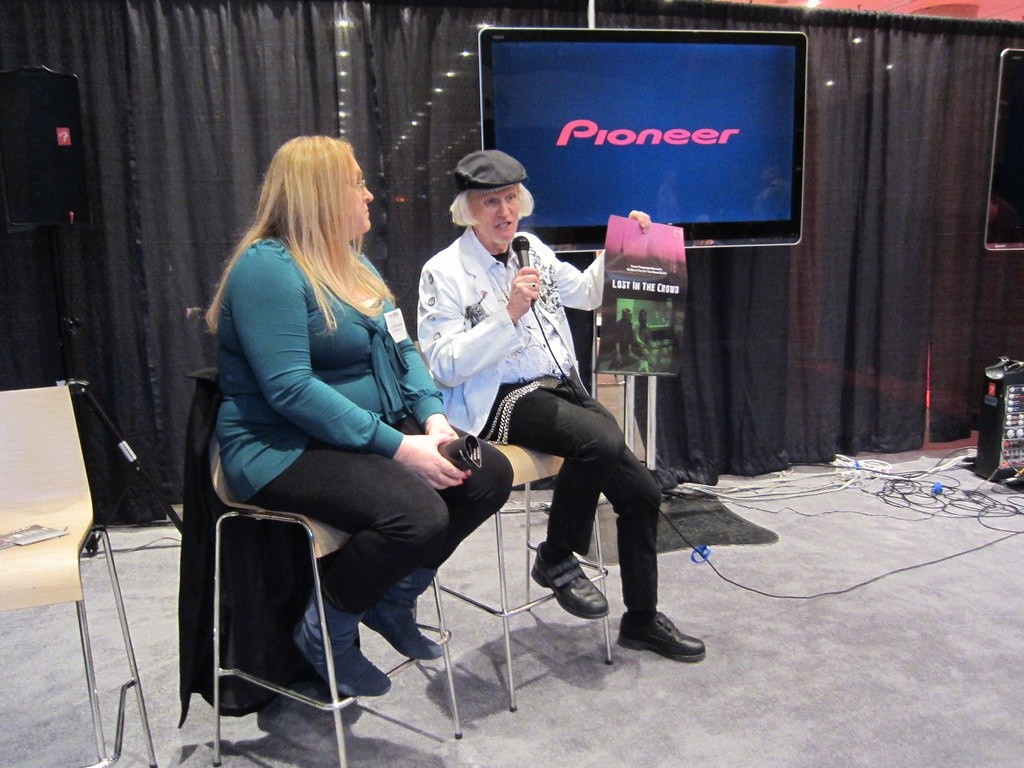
[413,340,616,712]
[0,384,160,768]
[186,372,463,768]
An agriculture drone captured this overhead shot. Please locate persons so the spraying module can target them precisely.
[611,305,655,365]
[413,147,707,664]
[203,132,514,698]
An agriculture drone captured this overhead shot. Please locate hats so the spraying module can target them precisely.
[454,149,528,190]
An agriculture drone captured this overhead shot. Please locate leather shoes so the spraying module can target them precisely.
[617,613,706,662]
[530,542,610,619]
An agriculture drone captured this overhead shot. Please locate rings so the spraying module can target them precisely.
[530,283,536,289]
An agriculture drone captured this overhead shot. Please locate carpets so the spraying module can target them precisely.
[543,492,779,567]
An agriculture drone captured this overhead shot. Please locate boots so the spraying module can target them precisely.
[362,567,443,659]
[292,587,391,697]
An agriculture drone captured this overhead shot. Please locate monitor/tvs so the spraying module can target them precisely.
[478,27,808,251]
[983,48,1024,250]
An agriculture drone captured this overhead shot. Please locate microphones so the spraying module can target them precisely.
[512,236,533,308]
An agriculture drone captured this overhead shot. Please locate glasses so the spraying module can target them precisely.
[345,178,367,191]
